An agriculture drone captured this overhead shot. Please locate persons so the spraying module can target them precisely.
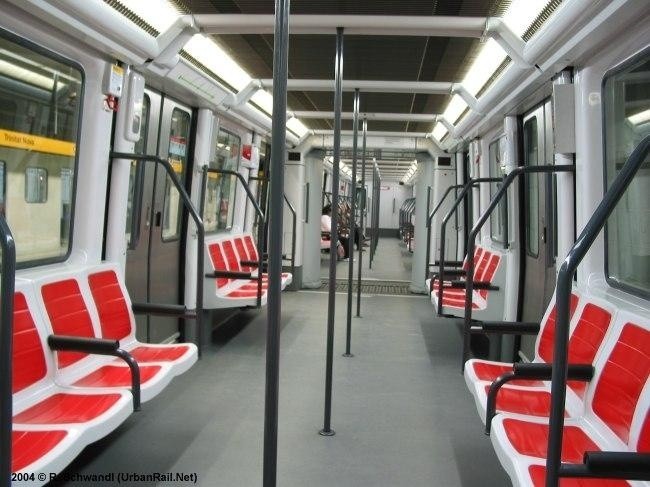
[321,201,371,263]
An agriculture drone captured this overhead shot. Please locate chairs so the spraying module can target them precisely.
[399,221,414,253]
[320,227,351,261]
[426,243,503,315]
[464,283,650,485]
[202,228,298,309]
